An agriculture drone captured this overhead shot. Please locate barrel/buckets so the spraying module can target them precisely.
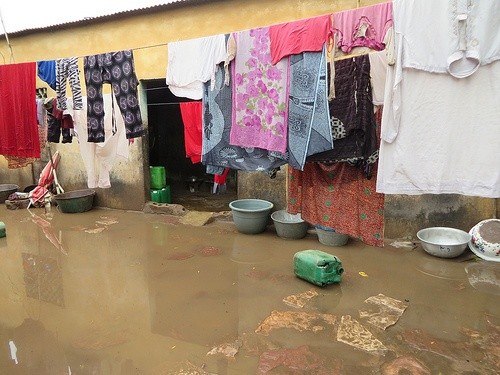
[293,249,344,287]
[150,167,171,202]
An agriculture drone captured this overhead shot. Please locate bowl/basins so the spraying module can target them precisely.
[468,219,500,262]
[316,227,351,247]
[229,199,274,234]
[417,227,471,258]
[271,207,307,240]
[55,189,96,214]
[0,184,18,203]
